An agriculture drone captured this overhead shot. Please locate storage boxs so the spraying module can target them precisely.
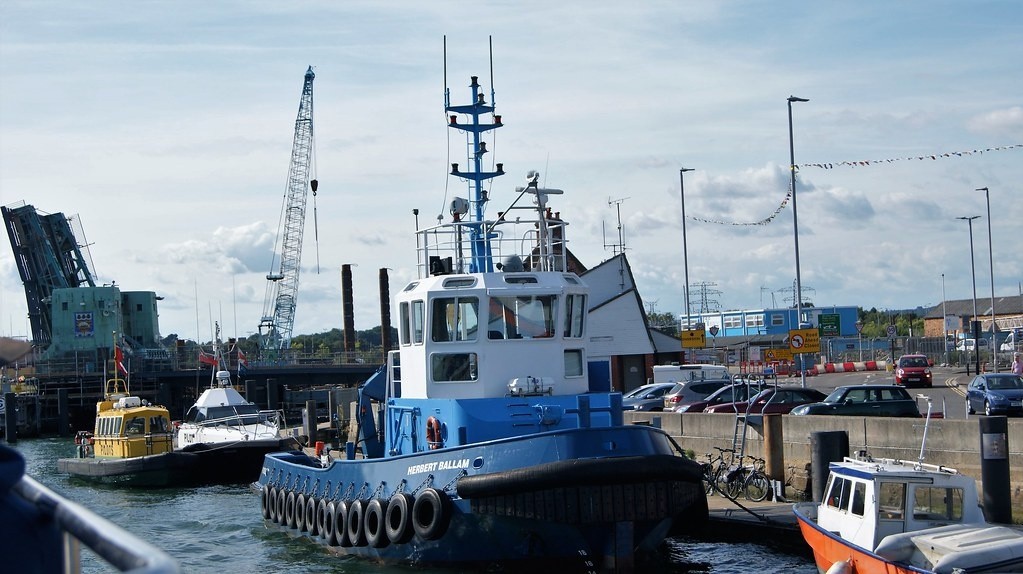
[508,376,555,395]
[120,396,141,407]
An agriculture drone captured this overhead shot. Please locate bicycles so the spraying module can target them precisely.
[696,446,770,503]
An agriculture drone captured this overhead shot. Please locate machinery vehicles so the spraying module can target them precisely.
[251,62,318,369]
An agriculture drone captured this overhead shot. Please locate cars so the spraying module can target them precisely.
[787,384,923,418]
[892,355,934,389]
[965,374,1023,416]
[617,376,758,413]
[704,386,829,414]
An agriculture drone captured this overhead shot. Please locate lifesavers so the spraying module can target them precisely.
[385,493,416,544]
[412,488,452,541]
[364,499,390,548]
[347,498,369,547]
[261,485,352,547]
[426,416,440,450]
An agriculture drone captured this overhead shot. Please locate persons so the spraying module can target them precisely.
[1011,355,1023,376]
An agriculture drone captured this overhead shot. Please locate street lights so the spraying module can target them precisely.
[974,187,999,374]
[787,95,808,385]
[955,216,981,376]
[680,166,697,361]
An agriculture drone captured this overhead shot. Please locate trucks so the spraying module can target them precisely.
[1000,330,1023,351]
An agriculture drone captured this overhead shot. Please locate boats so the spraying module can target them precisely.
[56,330,198,490]
[248,35,709,562]
[793,399,1023,574]
[175,275,290,482]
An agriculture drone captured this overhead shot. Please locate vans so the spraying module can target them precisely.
[652,363,732,382]
[956,337,989,350]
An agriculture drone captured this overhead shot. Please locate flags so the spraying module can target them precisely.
[239,348,248,370]
[115,343,127,377]
[198,346,219,366]
